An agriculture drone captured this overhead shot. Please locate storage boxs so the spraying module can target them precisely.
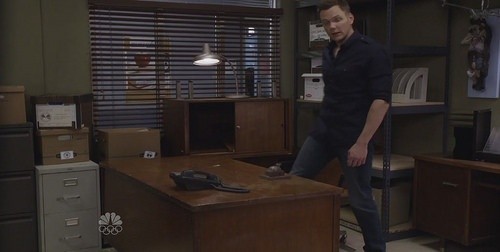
[368,181,411,227]
[36,126,90,164]
[96,128,161,167]
[34,103,81,128]
[0,84,28,126]
[300,19,330,102]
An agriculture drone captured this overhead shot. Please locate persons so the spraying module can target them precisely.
[289,0,392,252]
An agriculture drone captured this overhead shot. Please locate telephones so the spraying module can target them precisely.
[169,169,222,191]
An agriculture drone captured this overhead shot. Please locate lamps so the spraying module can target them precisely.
[193,43,250,98]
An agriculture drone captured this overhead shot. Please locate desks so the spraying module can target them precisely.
[99,156,344,252]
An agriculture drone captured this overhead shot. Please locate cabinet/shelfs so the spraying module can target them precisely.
[292,0,450,242]
[164,93,295,164]
[0,122,102,252]
[414,152,498,247]
